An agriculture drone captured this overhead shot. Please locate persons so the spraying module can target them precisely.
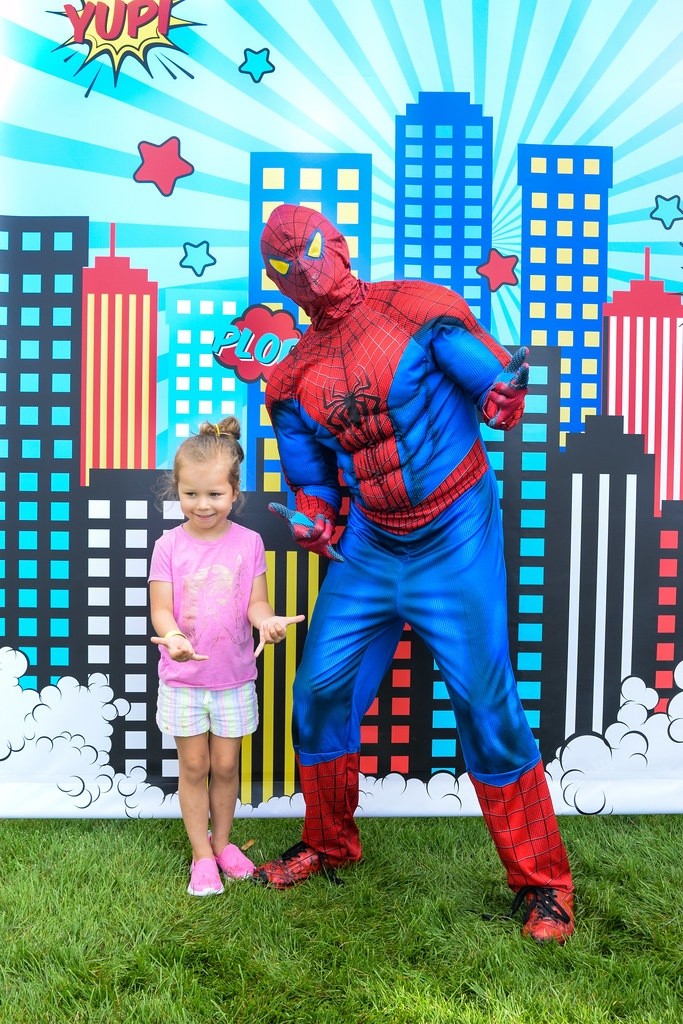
[260,205,578,941]
[145,417,306,896]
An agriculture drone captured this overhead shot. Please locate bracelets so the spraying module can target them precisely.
[164,630,188,639]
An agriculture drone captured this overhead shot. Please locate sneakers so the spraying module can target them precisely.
[252,840,345,889]
[186,857,225,896]
[481,885,574,944]
[208,834,256,882]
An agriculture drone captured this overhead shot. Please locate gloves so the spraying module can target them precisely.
[486,346,530,428]
[268,502,344,563]
